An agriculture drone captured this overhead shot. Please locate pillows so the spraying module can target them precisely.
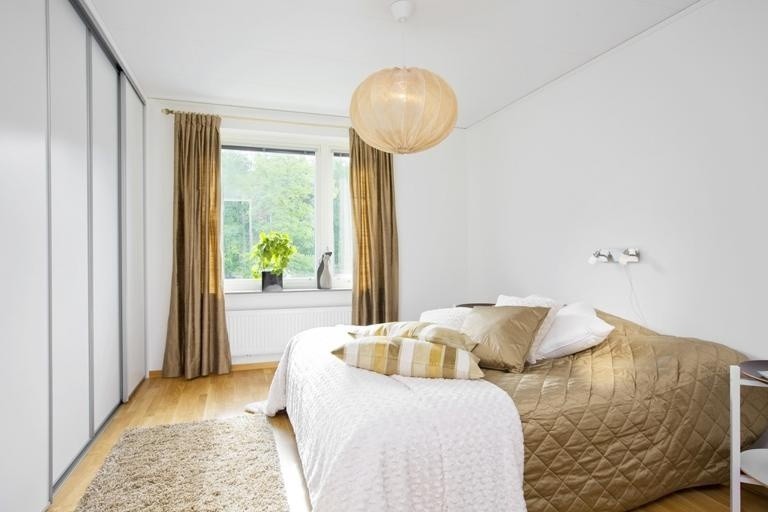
[496,294,539,306]
[348,321,478,351]
[461,307,550,374]
[398,338,485,379]
[536,301,616,360]
[420,307,473,332]
[524,295,567,363]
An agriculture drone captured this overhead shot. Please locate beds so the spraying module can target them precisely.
[245,293,768,512]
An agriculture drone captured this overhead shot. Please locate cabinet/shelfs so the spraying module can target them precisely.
[0,1,92,512]
[722,365,768,512]
[86,30,149,437]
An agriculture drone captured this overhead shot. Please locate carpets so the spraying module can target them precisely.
[75,414,288,512]
[332,337,399,379]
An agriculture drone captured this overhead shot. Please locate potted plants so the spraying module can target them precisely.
[251,230,297,294]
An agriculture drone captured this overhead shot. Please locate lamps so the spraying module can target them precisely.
[349,0,456,154]
